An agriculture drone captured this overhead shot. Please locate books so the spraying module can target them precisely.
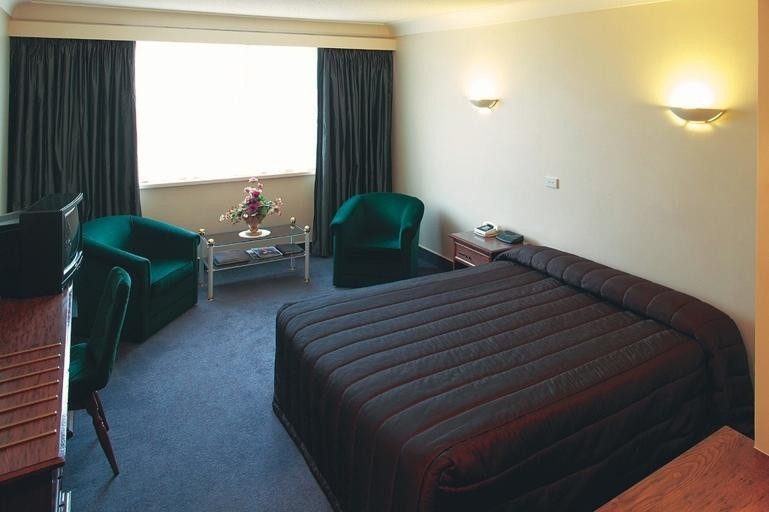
[213,247,251,267]
[273,244,305,256]
[244,246,283,261]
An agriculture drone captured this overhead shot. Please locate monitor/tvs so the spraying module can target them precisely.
[0,192,88,297]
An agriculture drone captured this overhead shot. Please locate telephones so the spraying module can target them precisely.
[473,221,502,237]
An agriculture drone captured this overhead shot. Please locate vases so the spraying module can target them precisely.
[242,217,264,235]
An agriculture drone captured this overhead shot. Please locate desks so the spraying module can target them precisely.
[0,279,75,512]
[591,425,769,510]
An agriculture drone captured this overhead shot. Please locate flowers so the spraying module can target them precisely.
[217,176,285,224]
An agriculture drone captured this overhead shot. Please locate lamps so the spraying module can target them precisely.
[468,98,498,106]
[669,107,726,122]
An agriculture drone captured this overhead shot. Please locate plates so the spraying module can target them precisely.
[239,228,271,238]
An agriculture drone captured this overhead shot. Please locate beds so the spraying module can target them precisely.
[271,244,752,512]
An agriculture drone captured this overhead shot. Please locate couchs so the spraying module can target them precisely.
[329,192,424,288]
[77,215,202,344]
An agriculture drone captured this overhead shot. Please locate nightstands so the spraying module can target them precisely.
[447,224,531,270]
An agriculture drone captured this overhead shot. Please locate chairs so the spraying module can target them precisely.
[69,265,131,475]
